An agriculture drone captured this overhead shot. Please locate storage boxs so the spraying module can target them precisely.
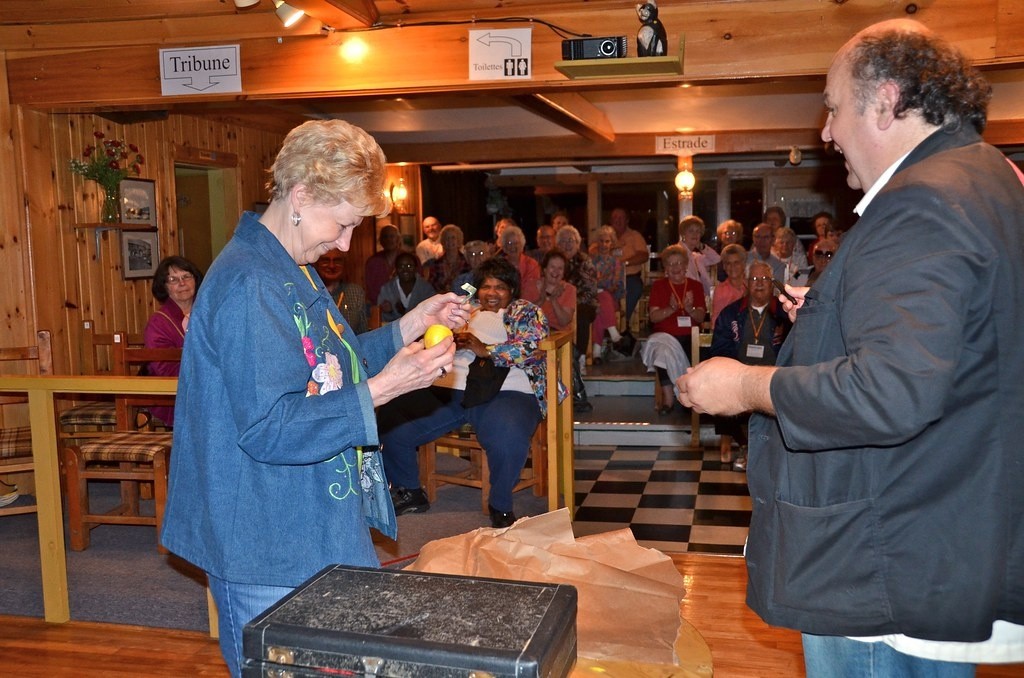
[243,564,579,678]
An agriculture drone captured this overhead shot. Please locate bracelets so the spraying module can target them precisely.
[625,260,629,267]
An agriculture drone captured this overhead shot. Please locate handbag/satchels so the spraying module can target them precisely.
[462,355,510,407]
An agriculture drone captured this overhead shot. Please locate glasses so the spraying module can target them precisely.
[721,231,736,236]
[471,251,484,256]
[749,276,770,281]
[167,273,193,284]
[667,262,683,266]
[399,264,413,269]
[814,249,833,258]
[505,240,521,245]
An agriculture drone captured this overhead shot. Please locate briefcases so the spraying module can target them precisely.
[242,566,578,678]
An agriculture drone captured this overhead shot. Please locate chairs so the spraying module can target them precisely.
[0,244,719,554]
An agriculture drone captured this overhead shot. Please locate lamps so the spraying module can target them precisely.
[381,177,408,201]
[673,170,695,200]
[790,146,801,164]
[233,0,305,27]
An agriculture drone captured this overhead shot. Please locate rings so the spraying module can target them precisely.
[466,320,469,324]
[440,367,447,378]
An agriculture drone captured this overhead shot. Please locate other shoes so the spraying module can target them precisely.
[720,442,732,462]
[659,404,674,416]
[579,359,587,377]
[733,444,747,472]
[613,336,630,350]
[573,402,592,412]
[594,357,603,364]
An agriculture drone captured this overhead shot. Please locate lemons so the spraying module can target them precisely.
[423,324,453,349]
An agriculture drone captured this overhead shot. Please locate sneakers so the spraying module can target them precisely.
[488,504,517,527]
[392,488,430,515]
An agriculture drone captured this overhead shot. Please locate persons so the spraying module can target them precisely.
[675,18,1024,678]
[646,207,848,471]
[143,256,207,427]
[318,209,648,531]
[158,117,473,678]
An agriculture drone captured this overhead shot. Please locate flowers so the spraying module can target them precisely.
[67,126,144,221]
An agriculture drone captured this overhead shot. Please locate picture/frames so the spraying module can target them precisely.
[119,177,159,280]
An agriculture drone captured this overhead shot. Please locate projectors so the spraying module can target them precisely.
[561,36,627,61]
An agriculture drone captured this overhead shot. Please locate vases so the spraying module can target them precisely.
[101,189,119,222]
